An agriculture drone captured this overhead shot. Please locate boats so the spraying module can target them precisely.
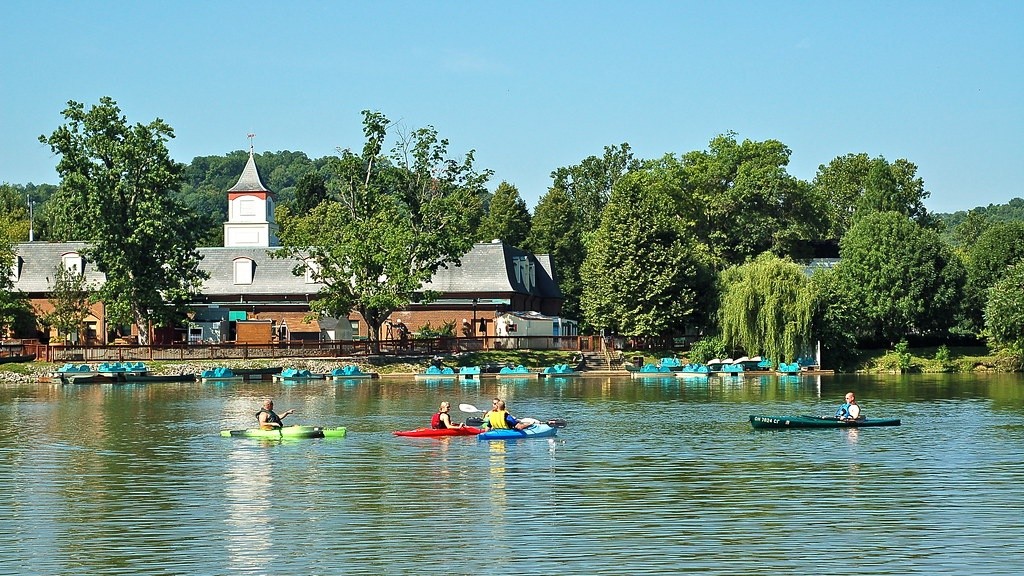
[631,364,674,377]
[656,357,684,371]
[332,366,372,380]
[122,362,155,376]
[201,367,243,381]
[775,363,802,376]
[220,424,346,441]
[673,363,715,377]
[0,343,36,364]
[271,368,324,382]
[392,422,485,438]
[90,363,131,377]
[413,366,458,379]
[749,414,901,431]
[48,364,94,377]
[707,359,733,371]
[495,365,540,378]
[477,418,558,440]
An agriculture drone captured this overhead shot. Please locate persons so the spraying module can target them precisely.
[477,398,533,430]
[432,401,465,429]
[823,392,860,422]
[256,399,296,430]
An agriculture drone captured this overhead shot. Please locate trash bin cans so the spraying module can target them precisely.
[633,357,643,367]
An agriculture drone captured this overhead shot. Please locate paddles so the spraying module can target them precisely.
[459,404,535,425]
[519,418,567,428]
[801,414,856,422]
[282,424,293,427]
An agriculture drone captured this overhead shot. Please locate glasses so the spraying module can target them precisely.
[845,398,849,399]
[493,402,497,405]
[268,403,273,405]
[446,406,450,408]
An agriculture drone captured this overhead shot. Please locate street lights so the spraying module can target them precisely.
[472,299,477,337]
[147,307,154,359]
